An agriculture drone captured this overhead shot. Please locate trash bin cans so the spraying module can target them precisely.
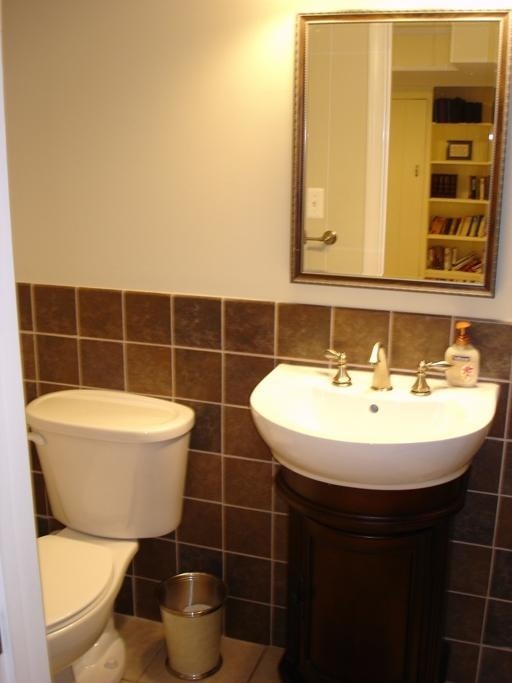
[156,572,227,681]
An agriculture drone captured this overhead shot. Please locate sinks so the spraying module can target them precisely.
[248,362,498,490]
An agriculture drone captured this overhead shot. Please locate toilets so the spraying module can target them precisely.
[24,391,195,682]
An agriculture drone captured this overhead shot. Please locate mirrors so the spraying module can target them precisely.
[288,7,512,300]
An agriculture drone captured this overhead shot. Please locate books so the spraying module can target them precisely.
[430,175,491,275]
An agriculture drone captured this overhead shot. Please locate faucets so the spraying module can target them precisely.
[367,341,391,390]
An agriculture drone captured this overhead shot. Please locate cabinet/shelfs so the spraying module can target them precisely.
[423,82,497,288]
